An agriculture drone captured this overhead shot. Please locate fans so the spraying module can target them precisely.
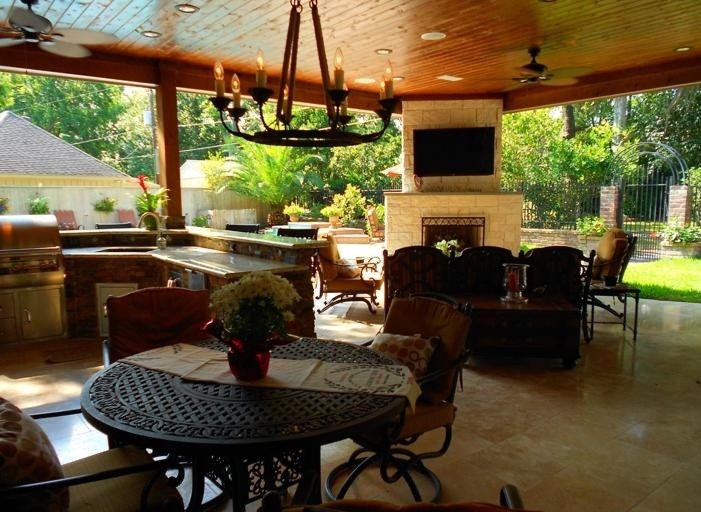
[0,0,116,60]
[484,46,593,96]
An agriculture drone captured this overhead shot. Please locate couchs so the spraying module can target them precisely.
[381,247,596,346]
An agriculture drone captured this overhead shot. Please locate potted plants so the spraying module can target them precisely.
[282,202,307,222]
[317,204,346,223]
[92,196,118,224]
[133,175,170,231]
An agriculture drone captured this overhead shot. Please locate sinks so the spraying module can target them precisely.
[99,247,155,252]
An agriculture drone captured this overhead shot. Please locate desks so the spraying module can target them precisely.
[80,333,412,511]
[456,284,582,369]
[584,275,642,342]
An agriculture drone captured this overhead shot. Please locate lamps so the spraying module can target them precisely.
[208,0,398,148]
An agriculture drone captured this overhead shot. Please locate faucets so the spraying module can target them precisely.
[136,212,166,250]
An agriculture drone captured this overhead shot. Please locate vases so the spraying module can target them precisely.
[219,332,270,379]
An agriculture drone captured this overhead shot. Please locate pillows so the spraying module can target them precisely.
[0,397,69,512]
[371,331,440,388]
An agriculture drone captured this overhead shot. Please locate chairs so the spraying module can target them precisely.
[259,483,525,512]
[100,286,220,363]
[583,227,637,322]
[313,236,383,315]
[324,288,471,505]
[0,392,228,512]
[278,228,318,242]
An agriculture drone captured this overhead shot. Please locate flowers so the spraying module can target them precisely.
[209,270,301,337]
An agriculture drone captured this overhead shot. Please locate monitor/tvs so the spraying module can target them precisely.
[413,127,495,176]
